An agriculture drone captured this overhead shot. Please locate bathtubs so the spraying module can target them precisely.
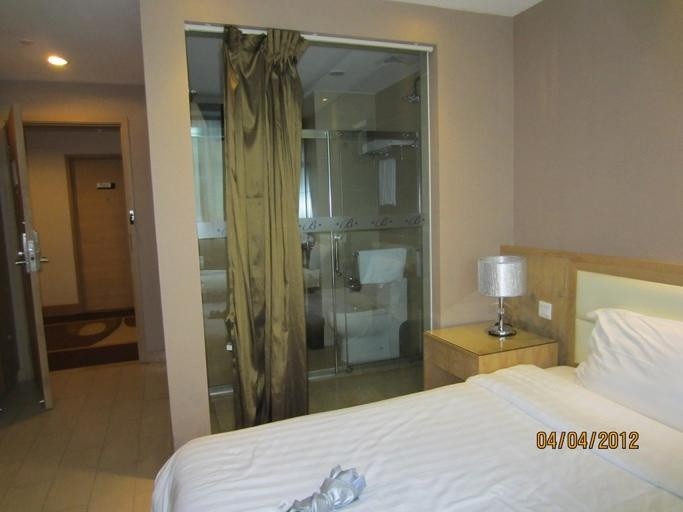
[200,265,321,296]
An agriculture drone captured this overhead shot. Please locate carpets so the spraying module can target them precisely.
[43,308,138,372]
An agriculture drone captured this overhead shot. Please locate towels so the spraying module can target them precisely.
[377,158,398,207]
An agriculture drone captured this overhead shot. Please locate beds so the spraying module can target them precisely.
[150,269,683,512]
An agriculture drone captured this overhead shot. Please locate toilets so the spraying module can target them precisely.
[327,276,409,363]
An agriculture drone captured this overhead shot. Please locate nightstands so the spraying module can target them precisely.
[423,323,558,390]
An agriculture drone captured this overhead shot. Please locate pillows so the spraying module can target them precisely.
[575,306,683,433]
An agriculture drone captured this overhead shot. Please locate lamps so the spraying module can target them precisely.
[477,255,526,337]
